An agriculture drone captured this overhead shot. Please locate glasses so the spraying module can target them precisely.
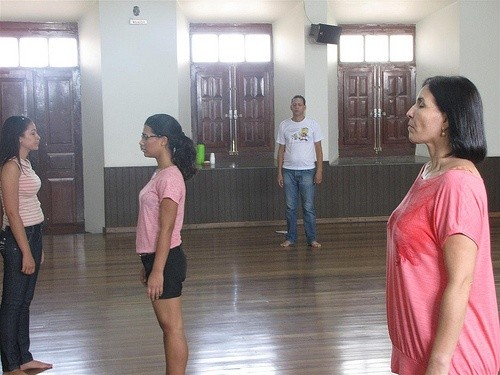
[19,114,28,131]
[141,133,165,140]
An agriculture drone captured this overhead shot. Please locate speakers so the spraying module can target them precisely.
[310,23,342,45]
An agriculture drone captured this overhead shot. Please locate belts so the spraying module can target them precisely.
[7,223,43,234]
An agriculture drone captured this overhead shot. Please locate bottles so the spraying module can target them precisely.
[210,153,216,164]
[195,143,204,164]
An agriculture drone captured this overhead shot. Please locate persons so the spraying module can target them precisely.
[0,115,53,375]
[387,76,500,375]
[136,113,197,375]
[276,95,323,247]
